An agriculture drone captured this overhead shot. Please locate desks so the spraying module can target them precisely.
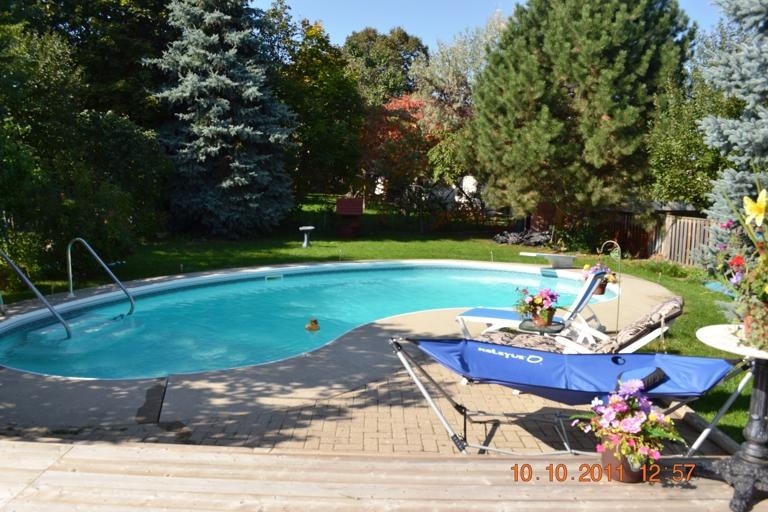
[695,324,767,511]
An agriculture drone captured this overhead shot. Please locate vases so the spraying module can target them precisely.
[599,446,651,484]
[593,282,606,294]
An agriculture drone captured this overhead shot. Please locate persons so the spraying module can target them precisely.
[306,318,318,335]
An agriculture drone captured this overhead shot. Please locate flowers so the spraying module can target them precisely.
[709,158,768,351]
[583,262,618,286]
[568,376,689,473]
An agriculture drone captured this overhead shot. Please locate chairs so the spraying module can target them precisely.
[453,268,686,352]
